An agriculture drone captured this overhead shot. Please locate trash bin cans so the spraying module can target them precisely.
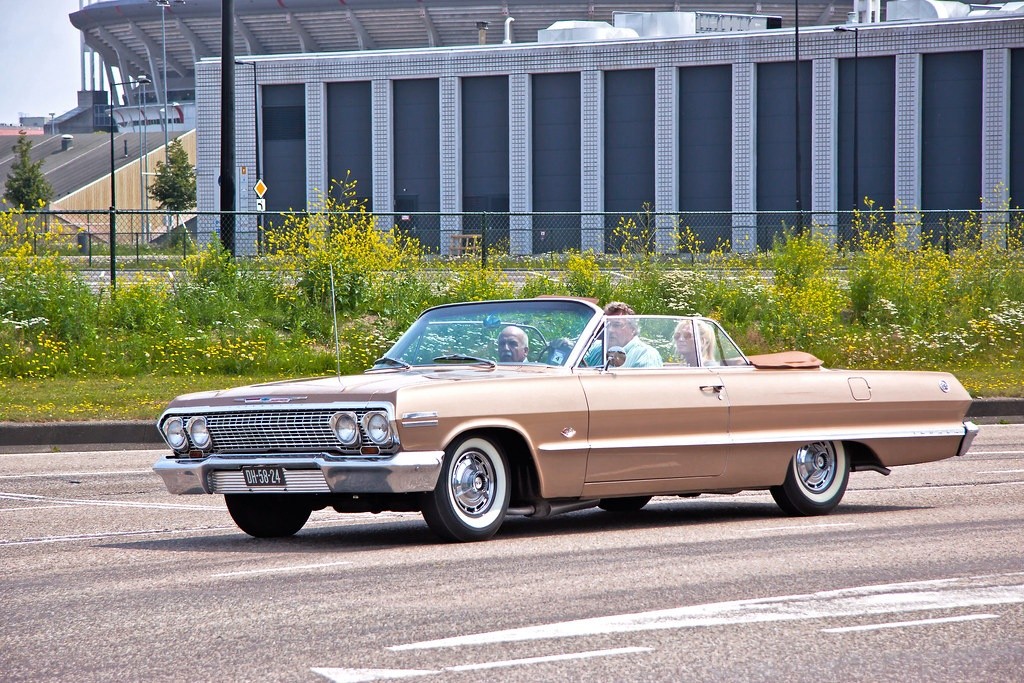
[77,232,88,254]
[61,132,74,151]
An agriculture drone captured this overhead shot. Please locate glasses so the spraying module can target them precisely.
[673,331,692,340]
[611,322,631,328]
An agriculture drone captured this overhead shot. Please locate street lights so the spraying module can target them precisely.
[832,26,861,249]
[155,0,186,230]
[49,112,56,135]
[108,77,151,305]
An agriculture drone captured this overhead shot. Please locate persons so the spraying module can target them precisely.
[497,326,529,362]
[555,302,663,369]
[673,320,716,368]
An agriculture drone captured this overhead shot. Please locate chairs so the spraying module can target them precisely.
[546,337,575,366]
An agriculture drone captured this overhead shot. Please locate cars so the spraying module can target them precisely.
[150,294,978,545]
